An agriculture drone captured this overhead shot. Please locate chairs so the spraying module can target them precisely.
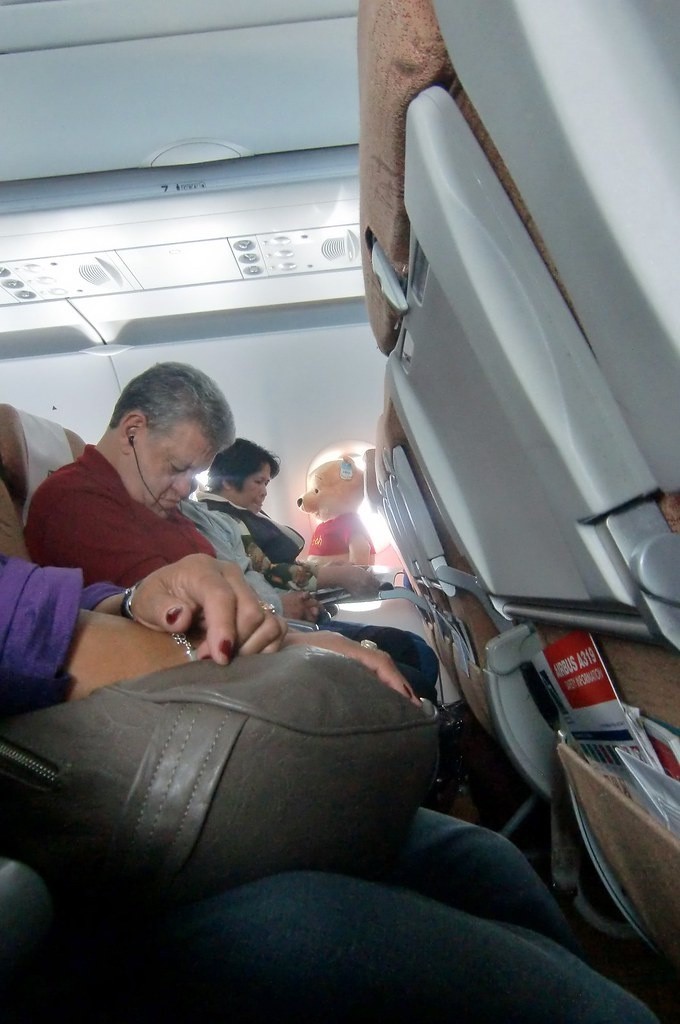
[0,0,680,982]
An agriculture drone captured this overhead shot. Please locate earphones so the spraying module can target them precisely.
[129,436,135,448]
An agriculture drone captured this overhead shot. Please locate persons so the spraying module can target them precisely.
[0,553,662,1024]
[196,438,380,599]
[23,361,422,709]
[177,478,440,702]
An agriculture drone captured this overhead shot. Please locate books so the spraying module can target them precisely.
[531,630,679,837]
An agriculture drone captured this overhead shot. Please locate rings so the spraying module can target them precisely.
[361,640,377,651]
[259,600,276,615]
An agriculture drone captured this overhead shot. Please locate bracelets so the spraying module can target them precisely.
[168,631,197,662]
[120,581,137,619]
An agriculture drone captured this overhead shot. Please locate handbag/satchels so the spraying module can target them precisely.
[0,654,443,915]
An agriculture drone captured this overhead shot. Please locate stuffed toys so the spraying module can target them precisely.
[296,456,377,566]
